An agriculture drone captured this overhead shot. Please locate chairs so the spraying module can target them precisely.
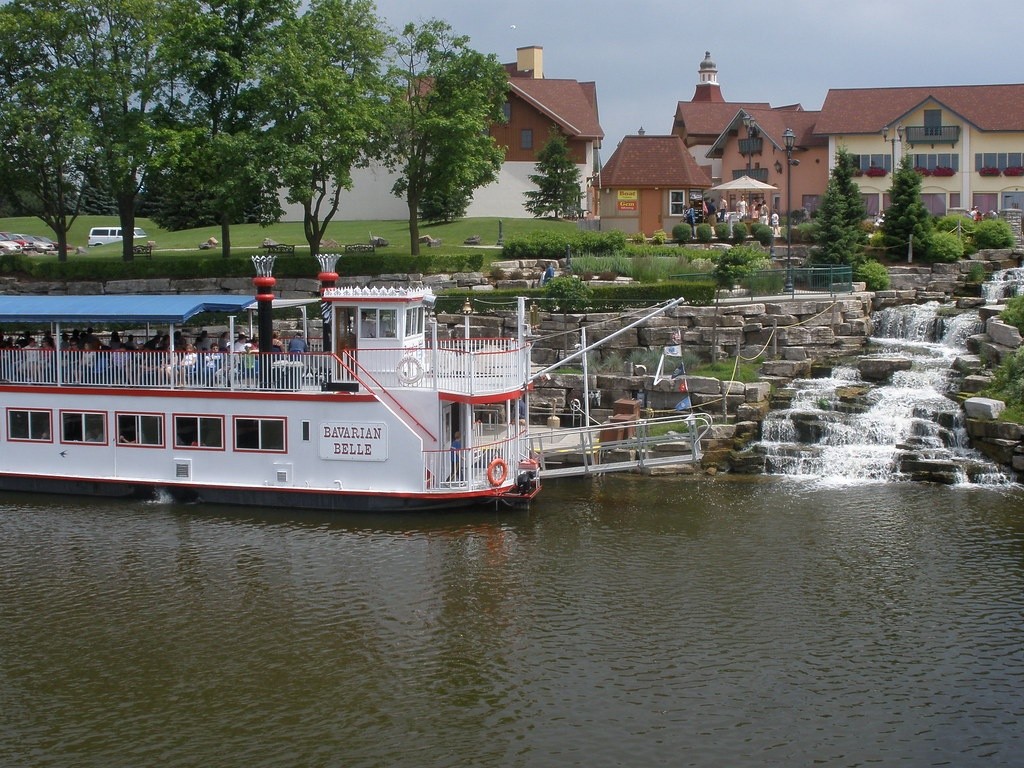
[727,213,742,235]
[757,216,769,226]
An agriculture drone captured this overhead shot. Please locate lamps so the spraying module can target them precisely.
[774,160,782,174]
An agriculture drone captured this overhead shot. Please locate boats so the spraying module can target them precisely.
[0,254,712,513]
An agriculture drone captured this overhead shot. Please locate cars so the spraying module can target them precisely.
[15,232,55,254]
[0,232,23,255]
[33,235,73,251]
[0,232,36,251]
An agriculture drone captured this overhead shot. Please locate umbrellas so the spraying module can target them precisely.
[711,173,779,193]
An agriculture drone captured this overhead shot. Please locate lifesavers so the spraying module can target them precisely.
[395,355,424,384]
[488,458,508,486]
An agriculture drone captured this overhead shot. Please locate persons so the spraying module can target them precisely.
[511,396,525,419]
[537,262,553,288]
[445,432,467,485]
[685,194,782,238]
[966,206,998,222]
[0,313,391,447]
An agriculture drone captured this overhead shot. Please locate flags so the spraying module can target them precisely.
[664,330,695,426]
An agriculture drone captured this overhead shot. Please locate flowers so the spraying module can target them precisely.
[1003,167,1024,176]
[978,166,1001,176]
[932,166,956,176]
[864,167,887,178]
[913,166,930,176]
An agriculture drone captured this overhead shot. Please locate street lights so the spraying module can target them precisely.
[781,125,796,294]
[742,111,756,210]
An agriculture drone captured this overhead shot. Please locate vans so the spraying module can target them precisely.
[88,227,147,247]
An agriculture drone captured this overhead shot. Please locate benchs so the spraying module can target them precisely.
[716,212,748,222]
[121,244,153,259]
[345,243,375,254]
[262,244,295,255]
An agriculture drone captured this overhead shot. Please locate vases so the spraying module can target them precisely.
[985,173,999,177]
[871,174,885,177]
[1005,172,1024,175]
[855,170,864,177]
